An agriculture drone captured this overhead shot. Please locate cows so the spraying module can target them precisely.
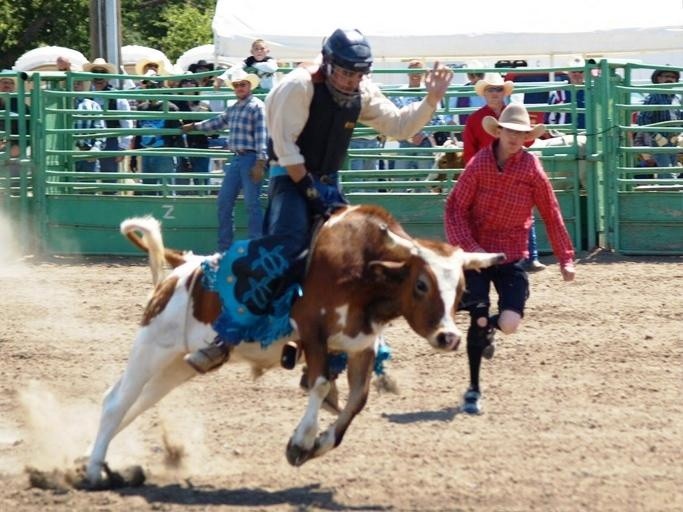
[81,203,507,490]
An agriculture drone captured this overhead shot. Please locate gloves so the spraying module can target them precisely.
[654,133,668,147]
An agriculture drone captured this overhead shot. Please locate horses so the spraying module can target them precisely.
[424,132,590,190]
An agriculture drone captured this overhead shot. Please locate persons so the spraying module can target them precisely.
[0,39,278,198]
[176,68,270,256]
[180,28,453,377]
[461,70,546,272]
[441,99,576,416]
[389,61,683,190]
[349,122,386,192]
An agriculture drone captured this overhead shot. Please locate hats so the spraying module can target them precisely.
[481,102,545,143]
[189,60,213,73]
[83,58,115,74]
[651,64,680,83]
[134,57,166,77]
[475,72,514,98]
[225,70,260,91]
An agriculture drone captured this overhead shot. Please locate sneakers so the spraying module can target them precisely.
[184,345,226,373]
[482,319,495,358]
[522,260,546,271]
[462,388,480,413]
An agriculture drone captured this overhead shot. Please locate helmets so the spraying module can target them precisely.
[319,28,372,101]
